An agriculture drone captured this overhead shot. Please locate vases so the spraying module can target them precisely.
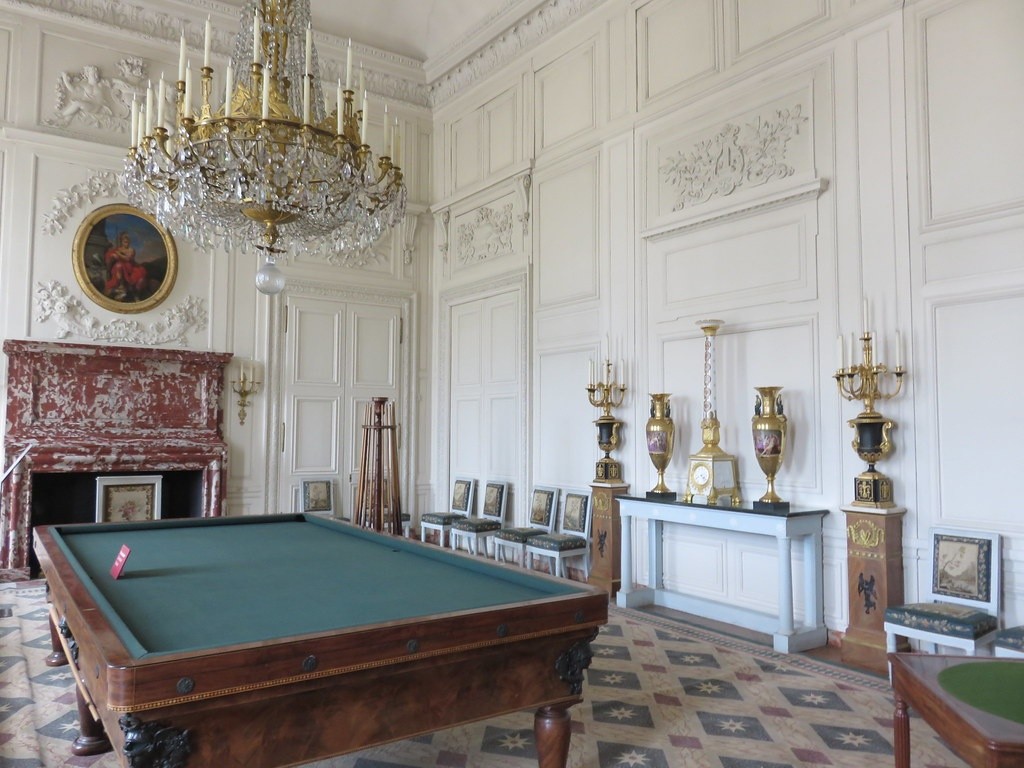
[752,386,786,503]
[646,393,675,494]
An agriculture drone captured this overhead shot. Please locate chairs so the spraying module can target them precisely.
[360,479,411,539]
[421,479,593,580]
[300,479,350,523]
[96,475,161,523]
[882,526,1024,687]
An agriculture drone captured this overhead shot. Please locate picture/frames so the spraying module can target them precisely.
[72,203,178,315]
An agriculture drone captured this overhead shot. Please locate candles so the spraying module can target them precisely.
[230,361,260,384]
[895,331,901,368]
[871,332,877,367]
[839,335,844,369]
[588,333,625,390]
[849,332,854,369]
[863,299,869,332]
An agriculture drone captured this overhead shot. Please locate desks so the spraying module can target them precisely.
[616,493,831,655]
[885,652,1024,768]
[32,512,609,768]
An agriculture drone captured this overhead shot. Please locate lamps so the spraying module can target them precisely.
[118,0,407,296]
